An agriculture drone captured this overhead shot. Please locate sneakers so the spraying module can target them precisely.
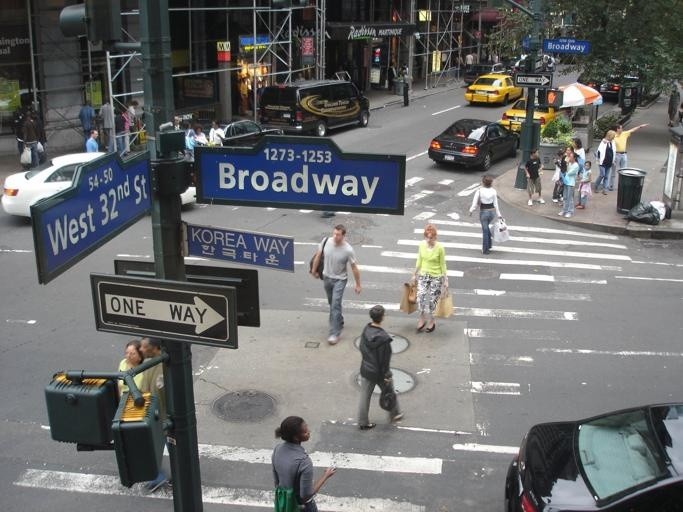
[528,198,545,206]
[327,335,338,344]
[144,480,173,493]
[552,197,585,217]
[594,185,613,195]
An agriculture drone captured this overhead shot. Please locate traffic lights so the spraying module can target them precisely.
[44,370,115,450]
[111,391,166,489]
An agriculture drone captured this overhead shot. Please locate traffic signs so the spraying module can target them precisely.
[193,134,406,215]
[513,72,552,88]
[30,149,154,286]
[520,35,531,50]
[542,36,591,55]
[89,271,238,350]
[186,224,294,272]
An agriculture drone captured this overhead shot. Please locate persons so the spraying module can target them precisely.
[310,224,362,345]
[667,84,681,127]
[524,124,650,218]
[454,52,478,77]
[11,105,48,171]
[469,176,501,255]
[77,99,147,156]
[174,115,226,162]
[116,339,144,397]
[237,73,267,117]
[358,305,404,430]
[388,63,410,107]
[271,416,337,512]
[412,224,449,332]
[140,337,173,493]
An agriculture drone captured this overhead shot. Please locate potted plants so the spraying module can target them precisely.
[538,112,575,169]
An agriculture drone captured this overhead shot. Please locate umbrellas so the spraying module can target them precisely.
[547,81,603,109]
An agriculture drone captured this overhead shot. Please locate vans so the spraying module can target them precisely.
[259,79,369,137]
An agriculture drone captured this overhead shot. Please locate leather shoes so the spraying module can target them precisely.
[392,412,403,421]
[361,423,376,430]
[417,322,435,332]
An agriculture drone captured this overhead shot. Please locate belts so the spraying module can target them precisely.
[616,151,627,154]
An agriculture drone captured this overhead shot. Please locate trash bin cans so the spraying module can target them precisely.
[616,167,648,215]
[392,76,405,95]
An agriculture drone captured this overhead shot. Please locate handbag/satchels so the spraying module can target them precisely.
[400,280,416,315]
[274,486,299,512]
[309,254,324,273]
[379,390,395,409]
[432,287,452,318]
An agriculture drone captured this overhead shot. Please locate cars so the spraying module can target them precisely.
[176,114,283,188]
[0,151,107,225]
[464,62,511,85]
[503,401,682,511]
[577,65,614,92]
[501,97,566,135]
[600,67,653,102]
[464,74,523,106]
[427,119,517,172]
